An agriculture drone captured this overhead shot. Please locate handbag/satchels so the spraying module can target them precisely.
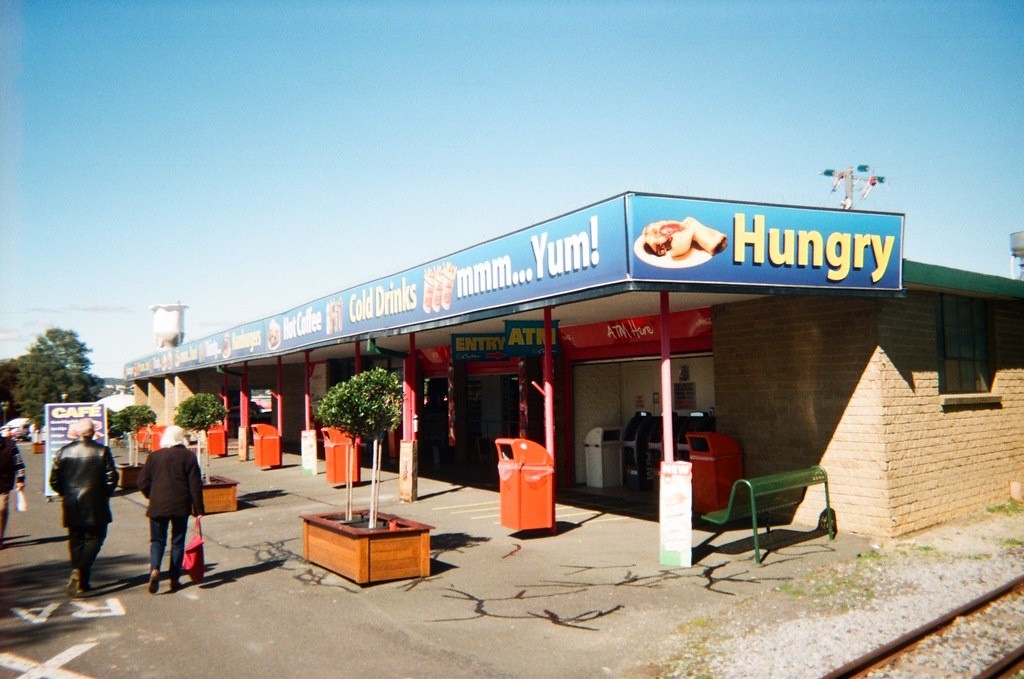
[182,515,205,581]
[12,489,27,512]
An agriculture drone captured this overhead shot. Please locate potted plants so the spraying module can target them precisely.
[298,367,436,584]
[172,393,240,515]
[112,405,157,487]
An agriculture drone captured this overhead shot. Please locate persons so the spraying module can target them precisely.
[138,425,205,593]
[0,433,25,551]
[47,418,120,595]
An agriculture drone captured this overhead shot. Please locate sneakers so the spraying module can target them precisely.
[76,584,90,592]
[147,569,159,594]
[170,581,182,590]
[66,570,80,598]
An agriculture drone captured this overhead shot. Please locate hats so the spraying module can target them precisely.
[159,426,189,449]
[78,419,96,437]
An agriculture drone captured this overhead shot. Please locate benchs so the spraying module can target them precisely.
[692,465,833,568]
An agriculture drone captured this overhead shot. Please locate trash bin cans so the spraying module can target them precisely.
[584,425,624,488]
[149,425,160,451]
[495,438,554,530]
[686,432,743,513]
[251,424,281,466]
[207,424,226,455]
[321,427,358,484]
